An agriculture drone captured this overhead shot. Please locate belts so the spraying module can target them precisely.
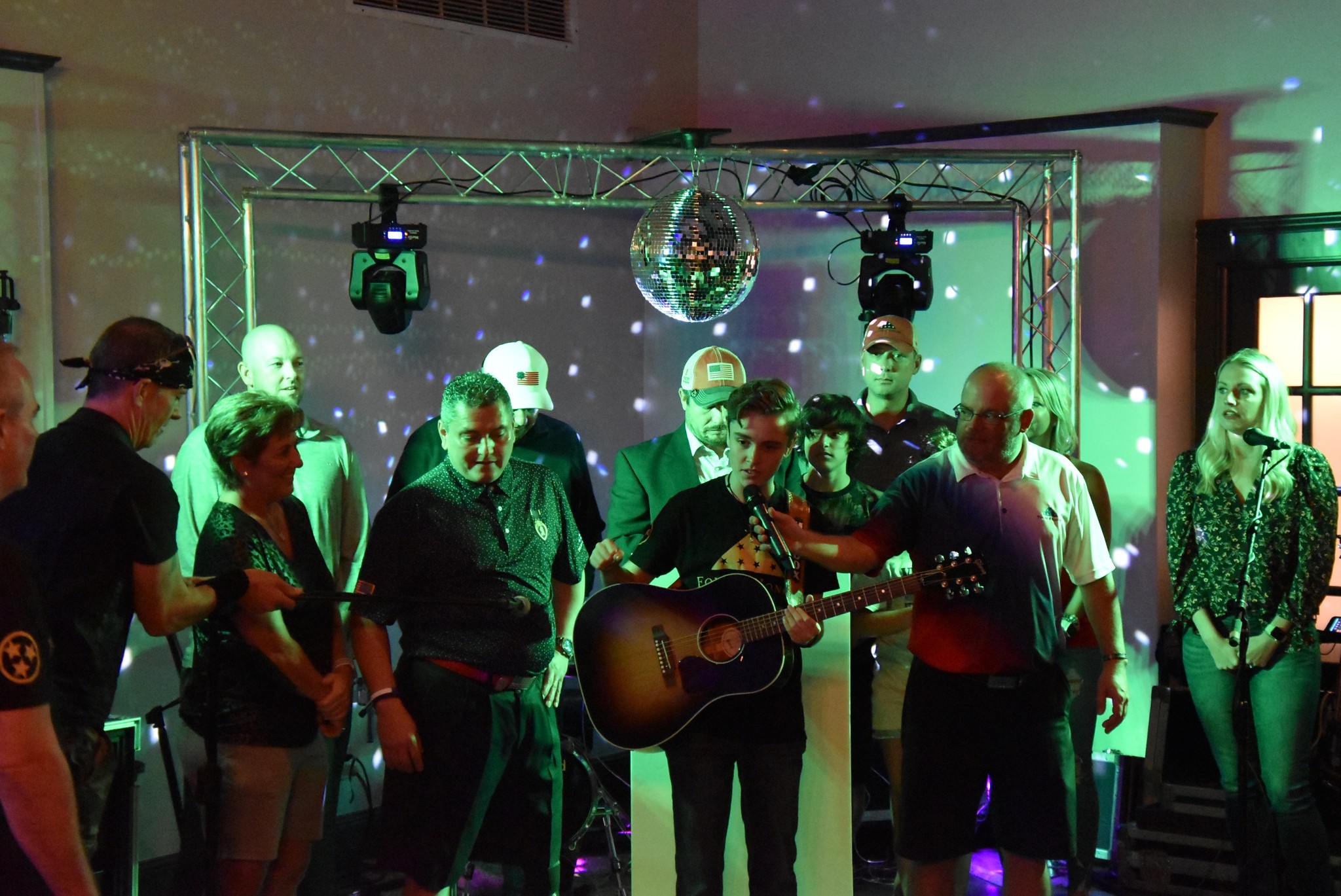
[421,657,548,691]
[960,672,1032,689]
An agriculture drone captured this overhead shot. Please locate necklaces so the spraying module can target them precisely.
[726,470,776,542]
[240,496,286,541]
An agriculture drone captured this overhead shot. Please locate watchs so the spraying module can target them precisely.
[791,634,818,648]
[556,636,574,658]
[1265,624,1287,643]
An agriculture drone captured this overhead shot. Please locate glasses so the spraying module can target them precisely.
[156,335,197,368]
[953,403,1025,425]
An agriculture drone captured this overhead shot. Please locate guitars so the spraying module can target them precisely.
[573,546,988,751]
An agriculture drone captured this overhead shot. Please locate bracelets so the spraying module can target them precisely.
[1102,653,1128,662]
[195,568,250,610]
[1061,612,1080,630]
[370,687,400,709]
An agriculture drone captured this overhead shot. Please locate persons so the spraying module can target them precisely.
[0,314,1129,896]
[1165,348,1338,896]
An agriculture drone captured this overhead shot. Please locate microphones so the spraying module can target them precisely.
[502,596,532,617]
[743,484,800,583]
[1242,428,1292,450]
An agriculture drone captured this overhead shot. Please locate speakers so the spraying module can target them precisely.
[1087,752,1145,859]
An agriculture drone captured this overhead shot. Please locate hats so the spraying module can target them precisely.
[681,345,746,407]
[861,315,919,356]
[482,341,553,411]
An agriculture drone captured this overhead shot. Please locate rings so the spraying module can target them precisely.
[342,728,346,732]
[1249,664,1254,668]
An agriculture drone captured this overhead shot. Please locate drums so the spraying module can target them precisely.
[558,738,599,856]
[581,700,631,827]
